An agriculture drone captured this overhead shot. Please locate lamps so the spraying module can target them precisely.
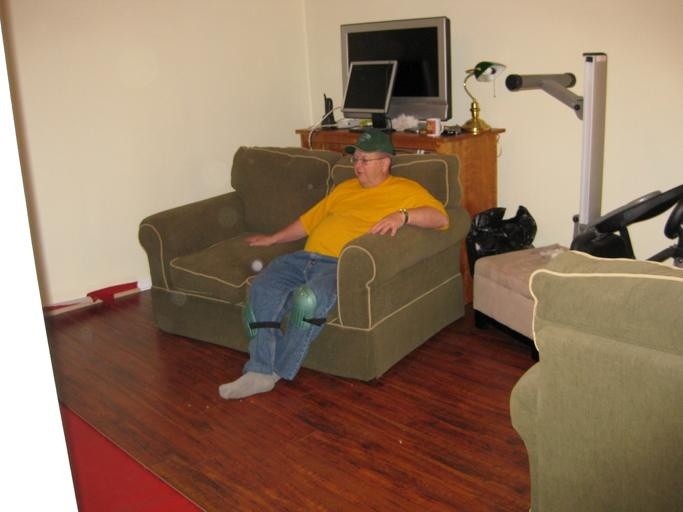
[461,59,507,134]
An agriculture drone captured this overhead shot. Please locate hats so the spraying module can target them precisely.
[345,129,395,157]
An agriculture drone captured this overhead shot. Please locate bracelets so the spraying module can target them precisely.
[397,208,409,224]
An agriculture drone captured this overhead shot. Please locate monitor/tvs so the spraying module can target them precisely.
[341,17,451,121]
[340,60,400,132]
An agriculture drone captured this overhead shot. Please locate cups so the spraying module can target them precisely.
[425,119,444,137]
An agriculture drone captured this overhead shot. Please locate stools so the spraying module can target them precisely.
[473,243,567,363]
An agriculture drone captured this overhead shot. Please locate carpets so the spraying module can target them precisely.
[58,398,206,511]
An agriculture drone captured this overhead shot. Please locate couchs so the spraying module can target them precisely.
[509,250,683,511]
[138,145,472,382]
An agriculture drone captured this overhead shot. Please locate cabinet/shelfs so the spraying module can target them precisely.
[296,124,506,308]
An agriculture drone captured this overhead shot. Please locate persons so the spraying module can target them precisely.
[216,126,451,398]
